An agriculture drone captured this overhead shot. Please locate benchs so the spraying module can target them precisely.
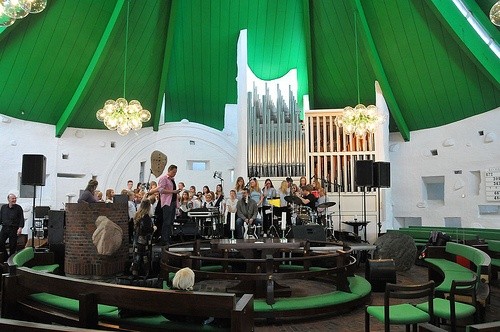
[0,225,500,332]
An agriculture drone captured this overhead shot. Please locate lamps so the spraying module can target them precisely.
[0,0,47,27]
[484,132,496,142]
[96,0,152,136]
[333,0,385,139]
[444,138,455,146]
[489,0,500,26]
[75,129,84,139]
[181,119,189,124]
[389,142,399,152]
[2,117,12,123]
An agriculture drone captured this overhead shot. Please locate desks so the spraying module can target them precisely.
[364,258,397,293]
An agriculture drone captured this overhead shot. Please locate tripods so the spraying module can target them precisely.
[266,197,280,239]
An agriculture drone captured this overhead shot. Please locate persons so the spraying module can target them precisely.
[131,199,157,278]
[157,165,179,246]
[77,179,98,203]
[0,193,25,278]
[95,176,326,240]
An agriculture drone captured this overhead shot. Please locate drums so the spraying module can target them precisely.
[318,215,332,227]
[297,207,312,220]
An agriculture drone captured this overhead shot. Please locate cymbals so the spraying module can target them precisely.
[318,202,336,208]
[284,196,302,204]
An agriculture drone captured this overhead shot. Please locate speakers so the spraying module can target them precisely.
[22,154,46,186]
[48,210,65,244]
[373,162,390,187]
[293,223,326,247]
[355,160,373,187]
[365,258,397,293]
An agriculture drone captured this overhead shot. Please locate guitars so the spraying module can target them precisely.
[243,193,258,240]
